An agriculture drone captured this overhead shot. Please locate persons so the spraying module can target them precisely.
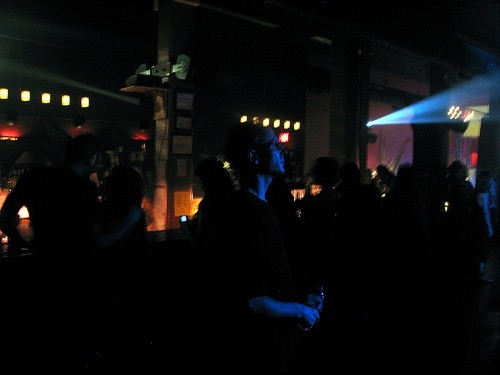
[0,120,500,375]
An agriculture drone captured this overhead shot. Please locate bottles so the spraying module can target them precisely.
[297,285,325,331]
